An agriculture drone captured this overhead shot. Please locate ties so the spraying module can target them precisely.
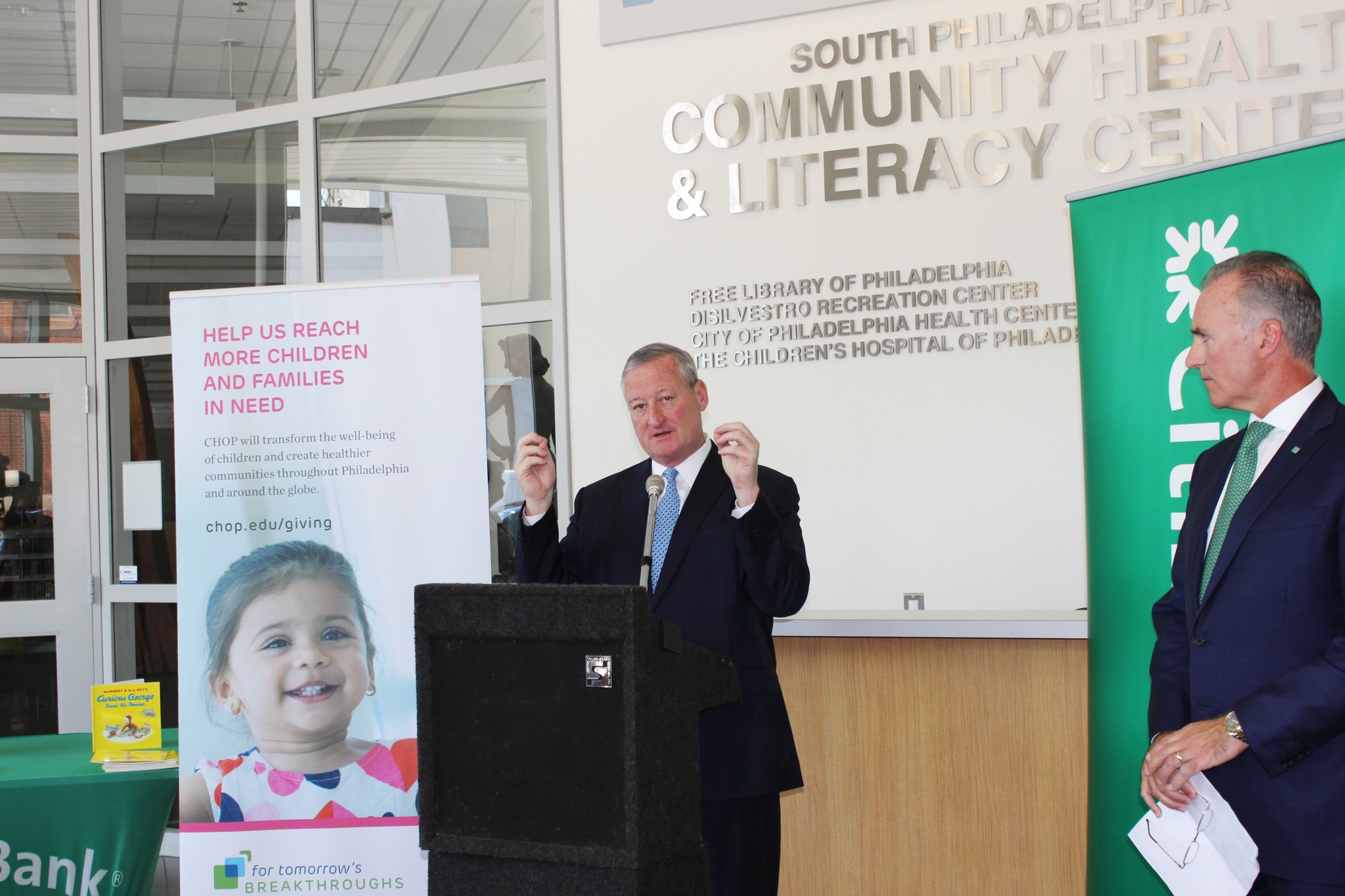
[1198,421,1275,607]
[650,467,680,595]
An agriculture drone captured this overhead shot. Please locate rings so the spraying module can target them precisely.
[1176,751,1186,766]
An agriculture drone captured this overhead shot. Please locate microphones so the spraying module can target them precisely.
[639,472,665,589]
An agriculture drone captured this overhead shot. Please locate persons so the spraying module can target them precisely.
[176,539,419,822]
[485,334,555,522]
[21,480,46,528]
[1139,249,1345,896]
[518,343,811,896]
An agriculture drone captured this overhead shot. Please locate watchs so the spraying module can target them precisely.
[1223,710,1250,745]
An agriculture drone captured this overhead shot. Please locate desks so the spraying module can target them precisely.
[0,728,178,896]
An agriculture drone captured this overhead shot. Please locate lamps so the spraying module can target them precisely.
[5,469,19,489]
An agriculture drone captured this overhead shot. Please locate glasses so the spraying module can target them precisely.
[1146,792,1213,869]
